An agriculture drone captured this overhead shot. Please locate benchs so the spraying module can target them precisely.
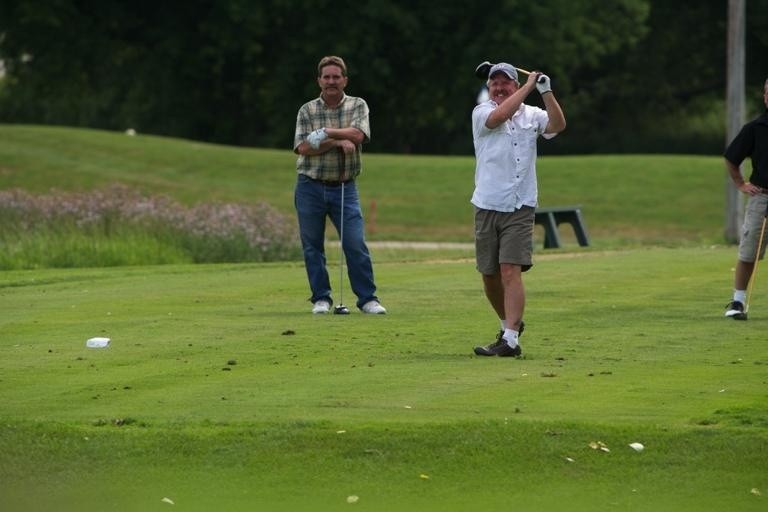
[534,204,591,247]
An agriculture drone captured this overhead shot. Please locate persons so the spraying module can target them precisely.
[470,62,566,357]
[293,56,386,315]
[724,79,768,316]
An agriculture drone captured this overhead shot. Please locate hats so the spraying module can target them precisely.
[488,62,519,81]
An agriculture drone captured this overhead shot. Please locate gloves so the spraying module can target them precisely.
[535,74,553,96]
[306,127,329,151]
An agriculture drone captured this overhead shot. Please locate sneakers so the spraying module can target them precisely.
[312,298,330,314]
[474,321,525,356]
[362,300,386,314]
[725,301,744,317]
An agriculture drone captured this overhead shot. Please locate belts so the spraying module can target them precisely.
[758,185,766,194]
[314,179,348,187]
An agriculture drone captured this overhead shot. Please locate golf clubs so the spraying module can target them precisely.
[474,60,547,85]
[334,147,351,315]
[732,200,767,320]
